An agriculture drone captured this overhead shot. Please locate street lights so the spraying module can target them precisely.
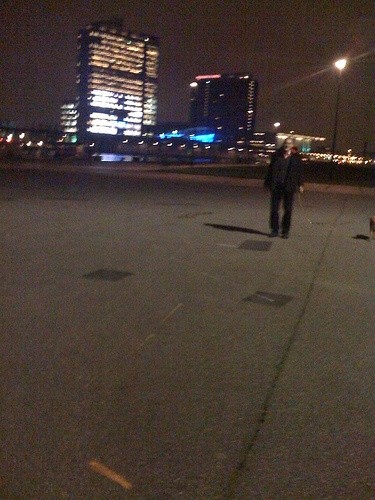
[330,58,347,153]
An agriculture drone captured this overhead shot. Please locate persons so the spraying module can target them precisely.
[263,136,304,239]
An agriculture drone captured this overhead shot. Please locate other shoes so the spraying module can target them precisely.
[282,233,288,238]
[270,231,278,236]
